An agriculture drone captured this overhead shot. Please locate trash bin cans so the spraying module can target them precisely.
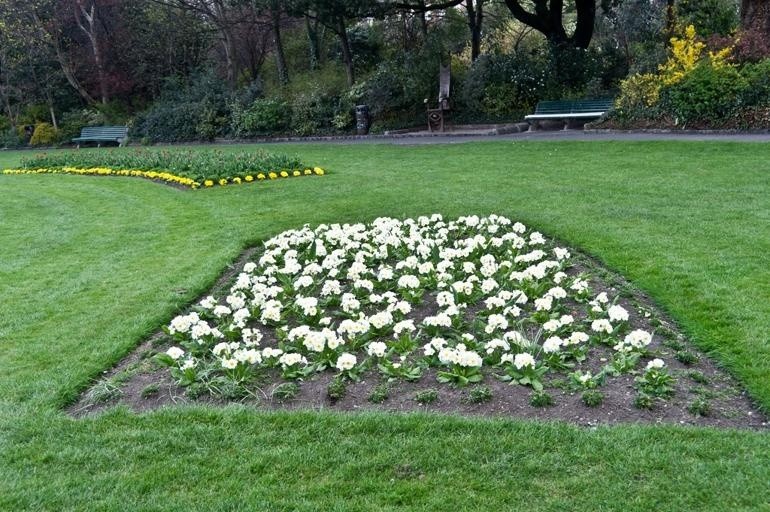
[25,125,34,146]
[355,105,369,134]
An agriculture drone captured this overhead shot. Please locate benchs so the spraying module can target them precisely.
[523,99,615,133]
[72,125,130,148]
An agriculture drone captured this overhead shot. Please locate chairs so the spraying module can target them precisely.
[423,60,455,133]
